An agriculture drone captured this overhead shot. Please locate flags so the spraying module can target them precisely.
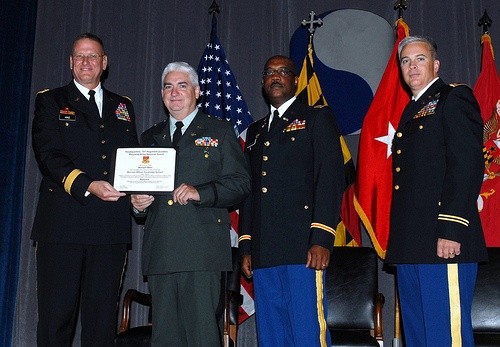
[197,17,255,324]
[293,43,362,247]
[471,33,500,248]
[354,20,411,259]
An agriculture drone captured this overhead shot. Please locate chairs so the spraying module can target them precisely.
[114,247,241,346]
[326,246,386,347]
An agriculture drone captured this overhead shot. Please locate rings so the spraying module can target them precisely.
[449,253,455,254]
[321,263,326,266]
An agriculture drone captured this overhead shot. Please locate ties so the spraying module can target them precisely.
[268,110,280,133]
[173,121,184,145]
[88,90,100,118]
[405,100,415,111]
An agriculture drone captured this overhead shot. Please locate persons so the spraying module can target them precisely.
[237,55,345,347]
[130,63,250,347]
[386,36,489,347]
[29,34,137,347]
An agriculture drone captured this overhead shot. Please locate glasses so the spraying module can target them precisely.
[263,66,297,77]
[72,52,104,60]
